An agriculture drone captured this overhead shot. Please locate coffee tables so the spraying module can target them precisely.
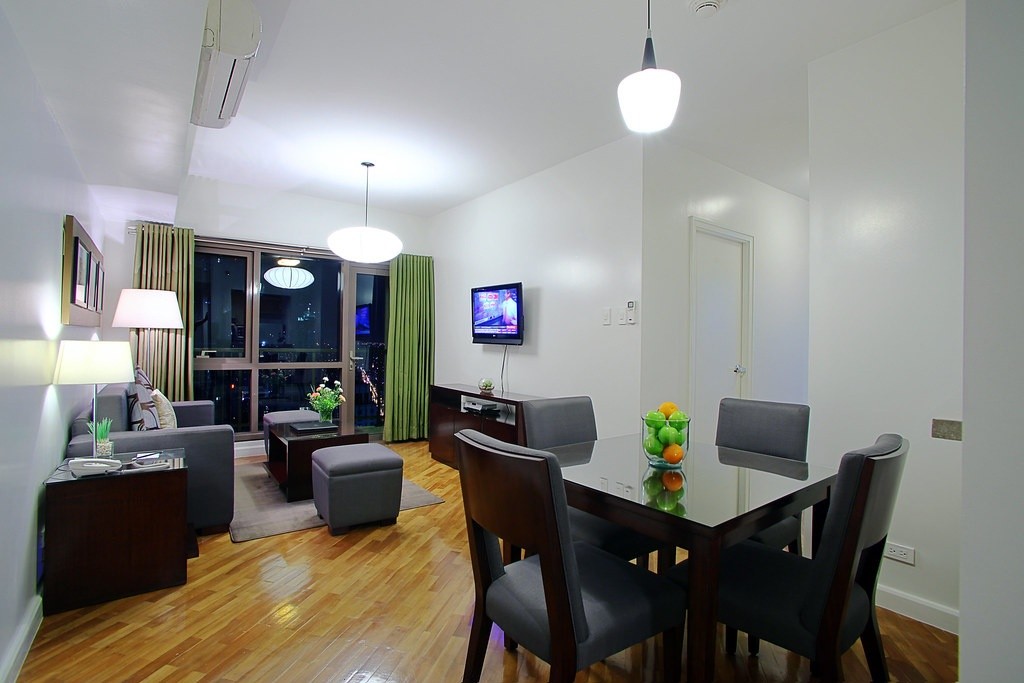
[263,420,370,502]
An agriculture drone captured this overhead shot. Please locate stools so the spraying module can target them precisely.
[262,410,319,456]
[312,442,404,535]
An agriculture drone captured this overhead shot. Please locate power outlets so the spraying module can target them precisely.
[882,542,915,565]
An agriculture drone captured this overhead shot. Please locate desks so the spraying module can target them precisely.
[503,432,838,683]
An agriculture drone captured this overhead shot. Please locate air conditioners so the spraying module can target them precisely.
[190,0,263,129]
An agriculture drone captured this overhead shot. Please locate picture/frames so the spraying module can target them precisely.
[72,236,105,313]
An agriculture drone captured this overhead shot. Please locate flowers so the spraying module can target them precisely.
[305,377,347,420]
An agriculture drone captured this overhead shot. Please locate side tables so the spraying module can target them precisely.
[42,447,188,616]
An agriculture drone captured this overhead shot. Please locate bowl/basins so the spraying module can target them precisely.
[641,414,691,470]
[642,461,688,517]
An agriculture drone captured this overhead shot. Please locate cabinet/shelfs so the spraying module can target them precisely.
[429,382,545,471]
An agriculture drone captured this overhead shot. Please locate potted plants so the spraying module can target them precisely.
[87,417,114,458]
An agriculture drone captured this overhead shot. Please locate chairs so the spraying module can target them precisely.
[454,395,910,683]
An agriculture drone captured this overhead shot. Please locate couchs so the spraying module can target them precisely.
[67,383,235,536]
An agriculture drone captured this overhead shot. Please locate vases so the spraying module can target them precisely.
[318,411,332,423]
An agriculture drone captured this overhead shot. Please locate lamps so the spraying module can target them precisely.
[52,333,135,458]
[618,0,681,134]
[325,161,402,263]
[111,289,184,379]
[263,259,315,289]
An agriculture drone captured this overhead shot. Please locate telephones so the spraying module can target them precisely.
[68,457,122,477]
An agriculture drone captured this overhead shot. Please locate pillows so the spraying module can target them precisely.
[127,383,161,431]
[134,363,153,391]
[150,389,177,429]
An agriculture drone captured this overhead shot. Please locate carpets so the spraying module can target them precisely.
[229,462,446,542]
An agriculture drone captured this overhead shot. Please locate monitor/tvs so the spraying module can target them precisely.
[471,282,523,345]
[355,303,373,340]
